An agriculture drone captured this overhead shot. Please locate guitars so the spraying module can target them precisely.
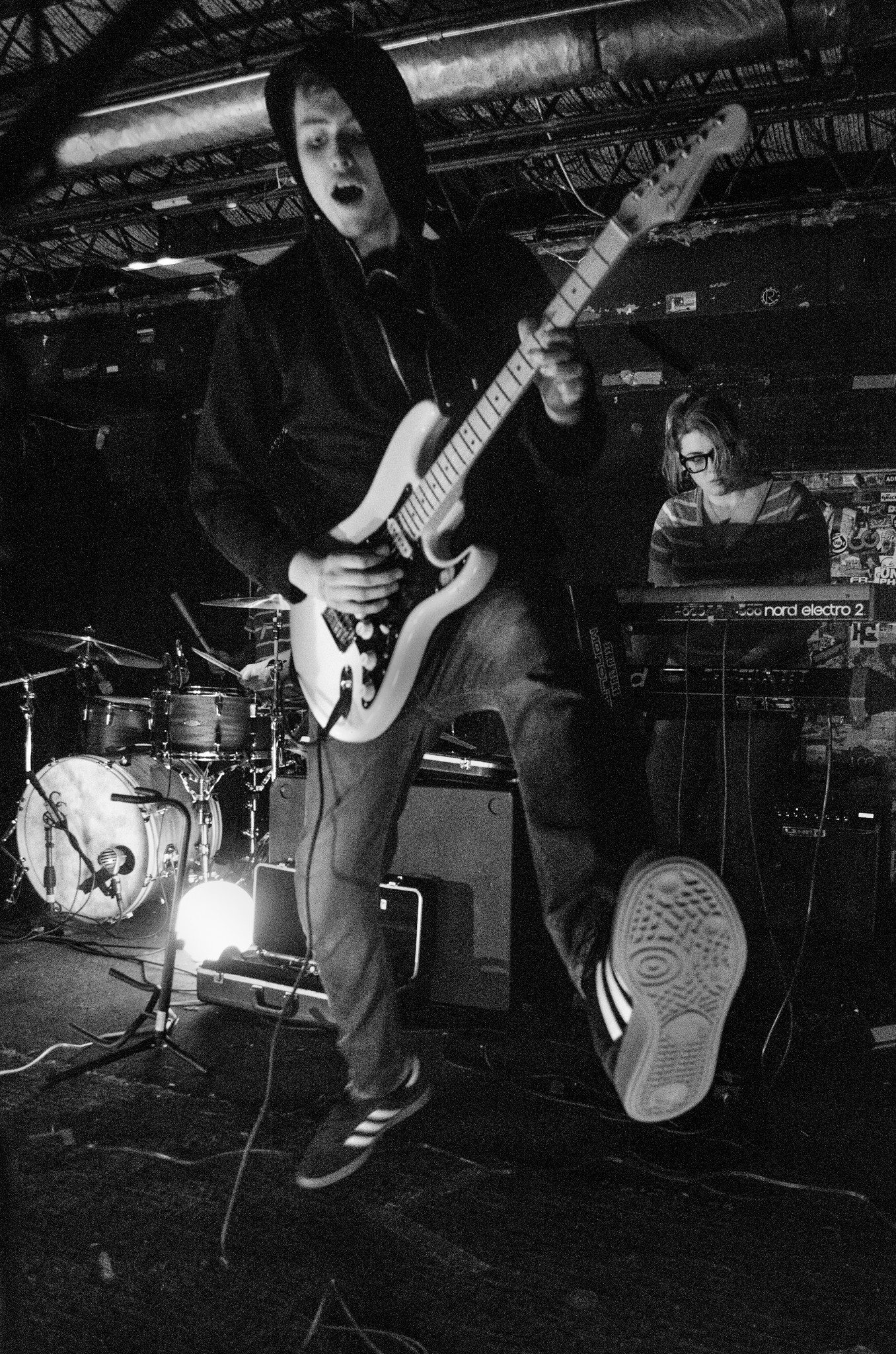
[279,86,759,745]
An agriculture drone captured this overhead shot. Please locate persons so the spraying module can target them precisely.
[187,34,751,1192]
[644,392,832,939]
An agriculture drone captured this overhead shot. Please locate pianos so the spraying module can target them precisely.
[619,569,896,927]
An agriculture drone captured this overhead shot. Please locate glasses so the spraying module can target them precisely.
[676,448,720,473]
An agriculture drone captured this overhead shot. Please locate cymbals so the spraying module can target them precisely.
[28,629,167,669]
[199,595,295,611]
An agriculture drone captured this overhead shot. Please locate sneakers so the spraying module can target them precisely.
[294,1055,430,1190]
[583,851,748,1122]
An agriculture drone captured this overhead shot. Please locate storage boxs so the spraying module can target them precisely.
[198,862,424,1025]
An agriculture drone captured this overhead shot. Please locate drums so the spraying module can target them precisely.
[73,690,156,755]
[156,680,276,765]
[12,740,225,927]
